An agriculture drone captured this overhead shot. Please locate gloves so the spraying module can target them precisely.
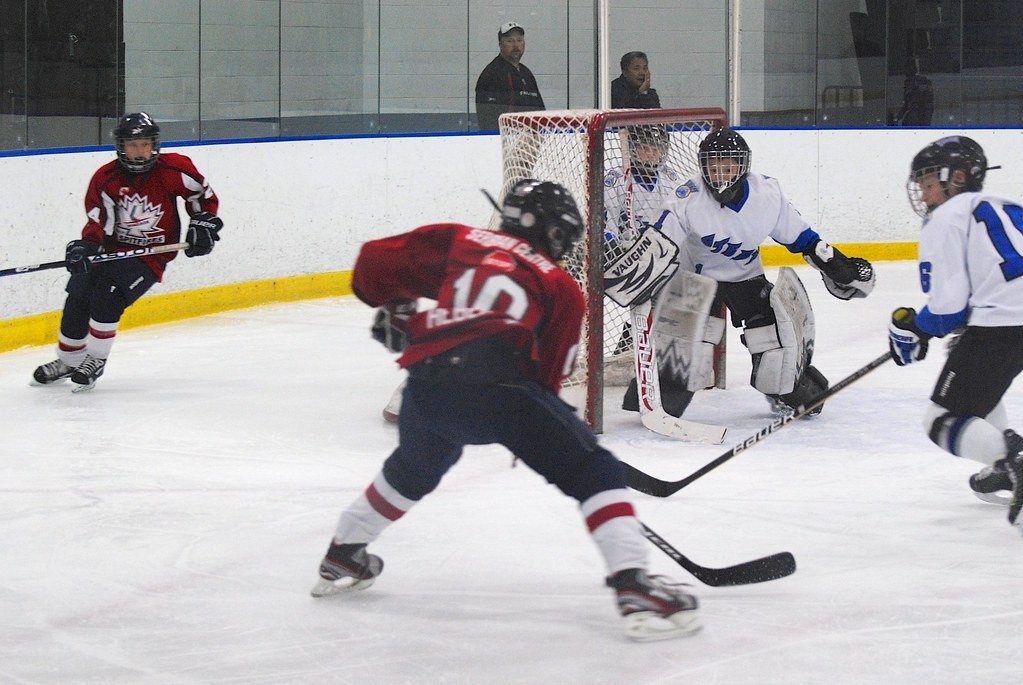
[65,240,94,283]
[888,306,932,366]
[371,302,418,352]
[184,210,223,258]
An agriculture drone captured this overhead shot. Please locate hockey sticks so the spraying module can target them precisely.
[618,128,730,445]
[635,520,796,586]
[617,349,893,497]
[0,242,190,277]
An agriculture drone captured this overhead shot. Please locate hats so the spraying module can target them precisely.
[498,21,525,39]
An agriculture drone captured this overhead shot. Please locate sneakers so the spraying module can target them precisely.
[969,462,1014,505]
[992,428,1023,525]
[310,539,383,596]
[28,357,75,387]
[605,567,704,642]
[71,354,106,393]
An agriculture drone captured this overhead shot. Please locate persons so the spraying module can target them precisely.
[569,124,683,386]
[887,135,1023,524]
[600,128,875,419]
[308,178,706,642]
[29,111,223,393]
[475,21,546,132]
[612,51,661,129]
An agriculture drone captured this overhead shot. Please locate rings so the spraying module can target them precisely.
[647,79,649,82]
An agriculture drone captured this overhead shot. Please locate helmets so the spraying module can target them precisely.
[497,178,585,262]
[698,128,752,202]
[906,135,987,218]
[628,124,669,174]
[110,111,160,173]
